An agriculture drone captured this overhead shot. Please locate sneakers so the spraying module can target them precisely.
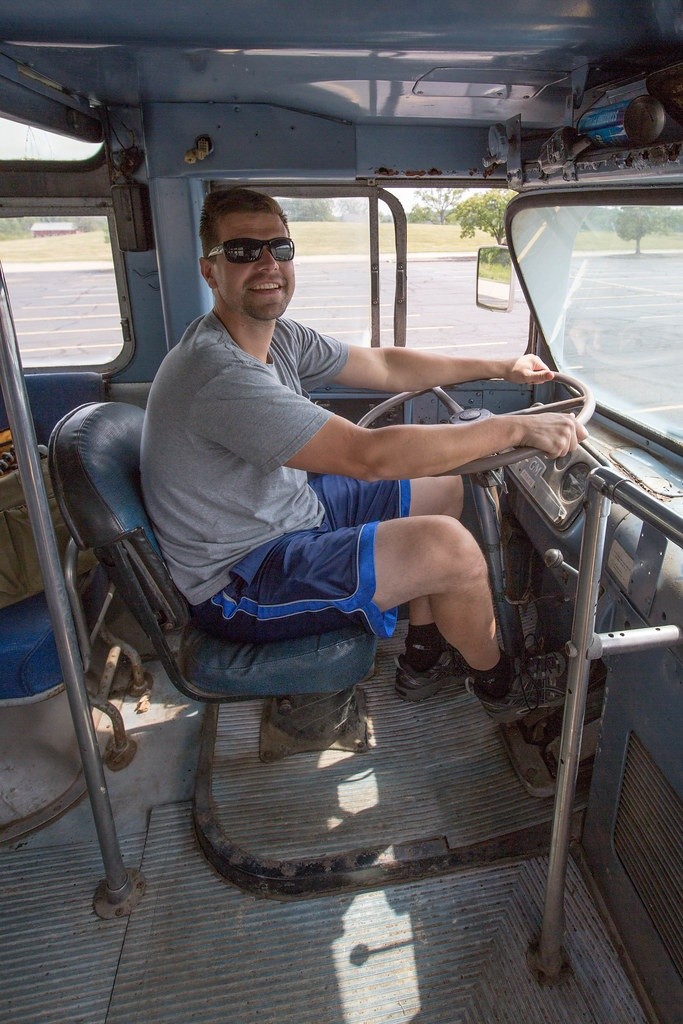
[394,644,482,703]
[478,650,606,723]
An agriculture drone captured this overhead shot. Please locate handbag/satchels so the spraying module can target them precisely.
[0,445,100,611]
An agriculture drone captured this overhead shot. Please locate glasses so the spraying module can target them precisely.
[207,237,295,265]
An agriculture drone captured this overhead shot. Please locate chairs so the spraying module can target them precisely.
[0,373,376,769]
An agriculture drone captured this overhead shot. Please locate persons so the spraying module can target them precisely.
[142,187,606,723]
